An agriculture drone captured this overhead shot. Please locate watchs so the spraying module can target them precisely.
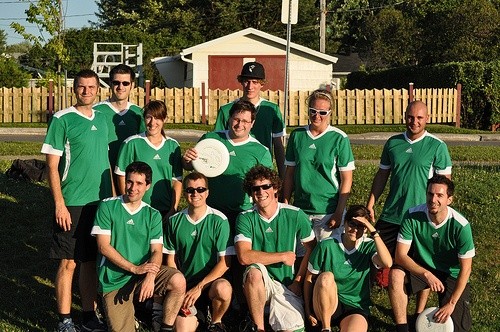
[371,230,380,236]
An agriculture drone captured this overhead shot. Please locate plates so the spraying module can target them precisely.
[191,138,230,177]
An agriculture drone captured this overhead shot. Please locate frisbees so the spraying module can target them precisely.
[415,307,454,332]
[191,138,231,178]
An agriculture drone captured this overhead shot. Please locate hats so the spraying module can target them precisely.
[241,62,265,79]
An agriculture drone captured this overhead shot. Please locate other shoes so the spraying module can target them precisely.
[53,317,105,332]
[152,308,163,332]
[205,319,225,332]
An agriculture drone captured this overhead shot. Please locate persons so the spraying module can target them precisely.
[234,164,315,332]
[114,100,183,310]
[90,162,186,332]
[304,204,393,332]
[213,62,287,201]
[41,69,117,332]
[282,89,355,272]
[184,101,274,328]
[162,173,232,332]
[92,64,148,195]
[366,101,452,326]
[389,175,476,332]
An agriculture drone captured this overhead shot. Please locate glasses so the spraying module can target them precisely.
[184,186,208,193]
[110,81,131,87]
[308,107,331,116]
[252,184,274,192]
